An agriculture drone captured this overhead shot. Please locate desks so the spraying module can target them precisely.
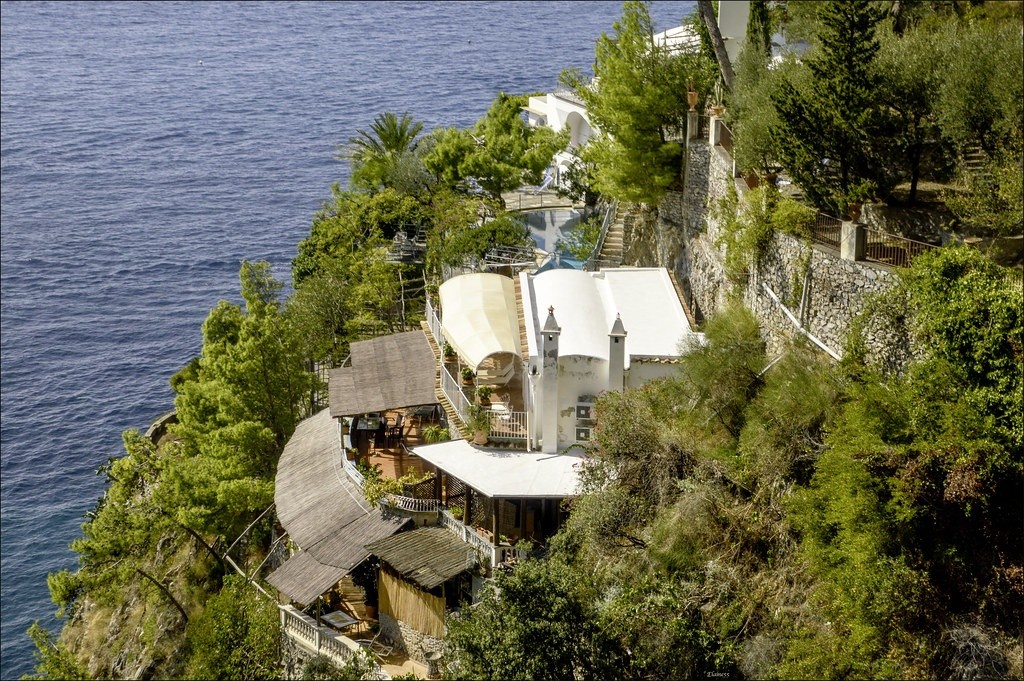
[320,610,360,640]
[357,417,382,446]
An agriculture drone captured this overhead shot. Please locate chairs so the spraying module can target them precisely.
[497,405,514,430]
[474,368,515,390]
[477,361,513,376]
[389,424,404,448]
[518,175,553,195]
[491,393,510,419]
[354,627,394,663]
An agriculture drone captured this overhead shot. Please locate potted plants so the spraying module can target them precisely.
[461,367,474,384]
[443,343,457,361]
[424,283,442,319]
[346,446,359,460]
[342,418,349,435]
[351,556,378,618]
[476,384,493,405]
[468,406,492,444]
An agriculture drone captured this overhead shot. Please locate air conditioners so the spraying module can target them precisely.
[575,425,595,444]
[576,402,598,421]
